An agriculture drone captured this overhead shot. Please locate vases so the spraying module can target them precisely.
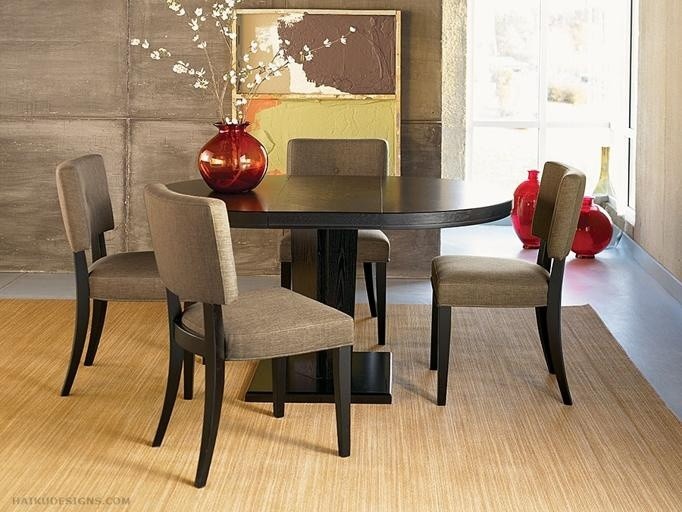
[198,122,268,194]
[511,170,540,249]
[571,198,613,260]
[592,143,617,220]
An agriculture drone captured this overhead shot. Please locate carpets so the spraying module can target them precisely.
[0,296,682,509]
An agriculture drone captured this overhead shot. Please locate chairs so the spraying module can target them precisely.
[429,161,587,406]
[279,138,390,346]
[55,153,199,399]
[142,181,356,489]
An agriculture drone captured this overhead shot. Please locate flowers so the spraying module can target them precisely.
[125,0,356,124]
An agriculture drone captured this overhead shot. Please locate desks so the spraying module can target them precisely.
[163,175,514,404]
